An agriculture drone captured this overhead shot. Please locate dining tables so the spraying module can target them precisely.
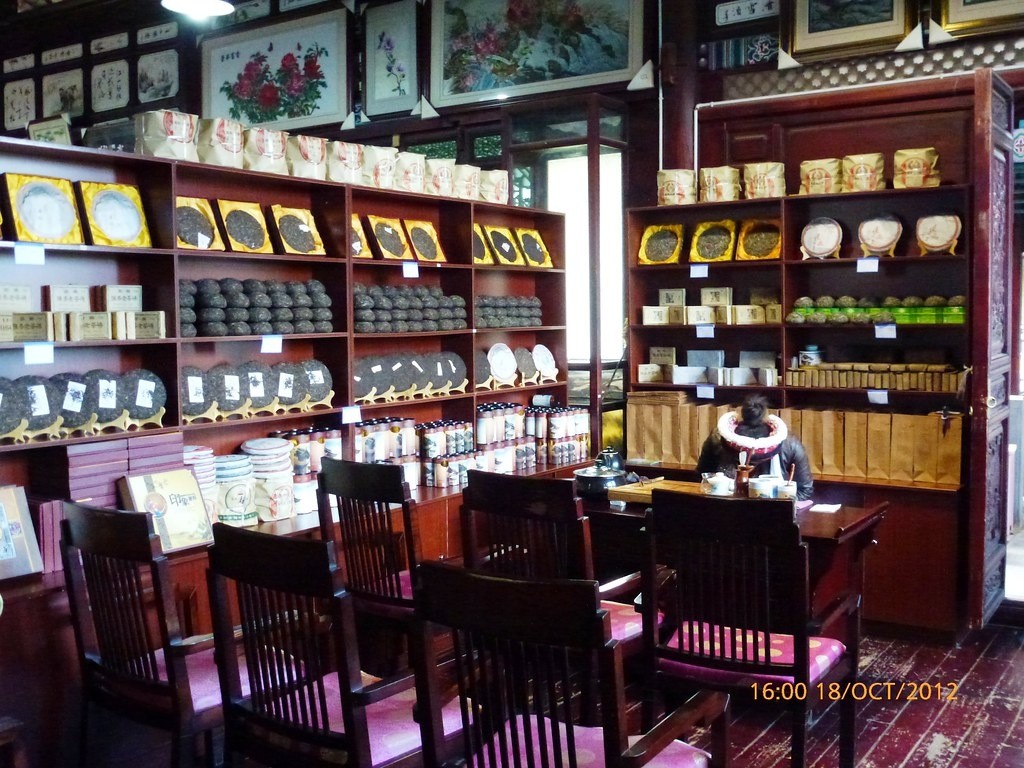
[583,478,891,711]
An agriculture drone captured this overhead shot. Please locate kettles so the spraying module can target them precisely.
[593,445,623,472]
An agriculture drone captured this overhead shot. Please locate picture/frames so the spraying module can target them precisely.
[421,0,654,119]
[81,120,135,152]
[196,0,355,134]
[778,0,924,71]
[25,113,74,145]
[360,0,421,122]
[928,0,1024,46]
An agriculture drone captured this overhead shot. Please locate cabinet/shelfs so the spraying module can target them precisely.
[350,179,597,587]
[626,184,975,631]
[0,136,353,768]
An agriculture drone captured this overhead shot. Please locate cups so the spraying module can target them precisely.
[747,471,798,499]
[701,472,722,495]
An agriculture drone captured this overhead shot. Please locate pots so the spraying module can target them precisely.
[574,460,625,500]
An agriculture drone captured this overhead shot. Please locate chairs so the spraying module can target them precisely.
[413,559,731,768]
[316,457,465,713]
[205,522,482,768]
[633,488,864,767]
[460,470,668,734]
[58,501,310,768]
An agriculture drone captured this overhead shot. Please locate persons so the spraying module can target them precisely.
[692,394,814,502]
